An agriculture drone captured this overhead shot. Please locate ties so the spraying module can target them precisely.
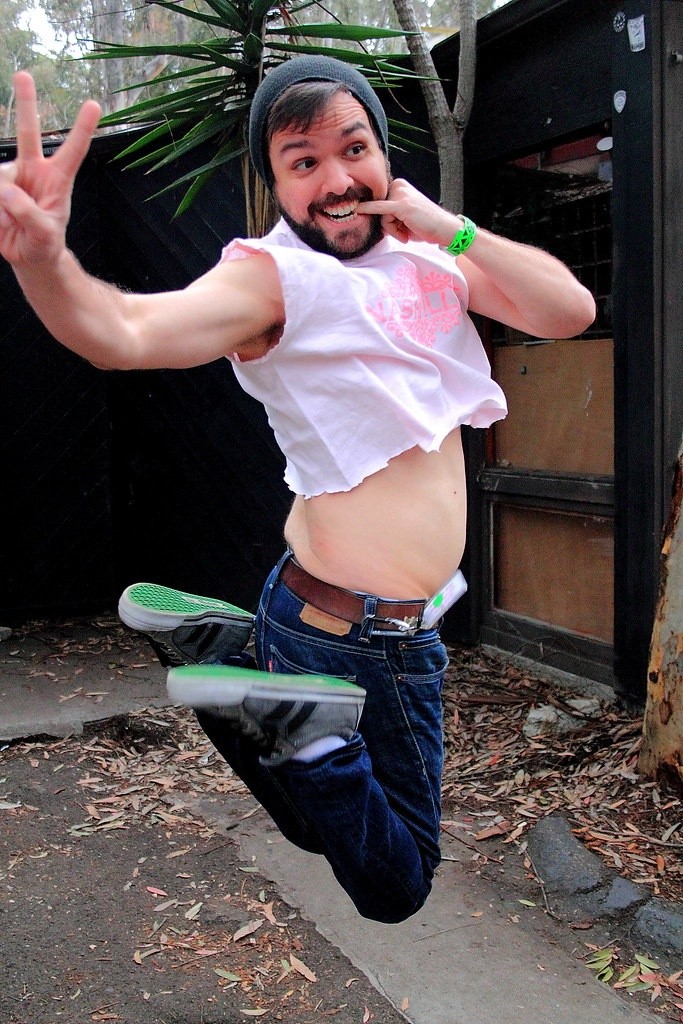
[248,55,389,195]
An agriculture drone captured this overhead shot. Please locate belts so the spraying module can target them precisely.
[281,558,441,631]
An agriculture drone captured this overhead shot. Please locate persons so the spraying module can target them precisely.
[2,54,597,925]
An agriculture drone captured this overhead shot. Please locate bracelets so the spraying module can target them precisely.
[439,213,478,258]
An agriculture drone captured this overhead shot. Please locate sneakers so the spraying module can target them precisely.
[116,583,258,668]
[166,658,368,767]
